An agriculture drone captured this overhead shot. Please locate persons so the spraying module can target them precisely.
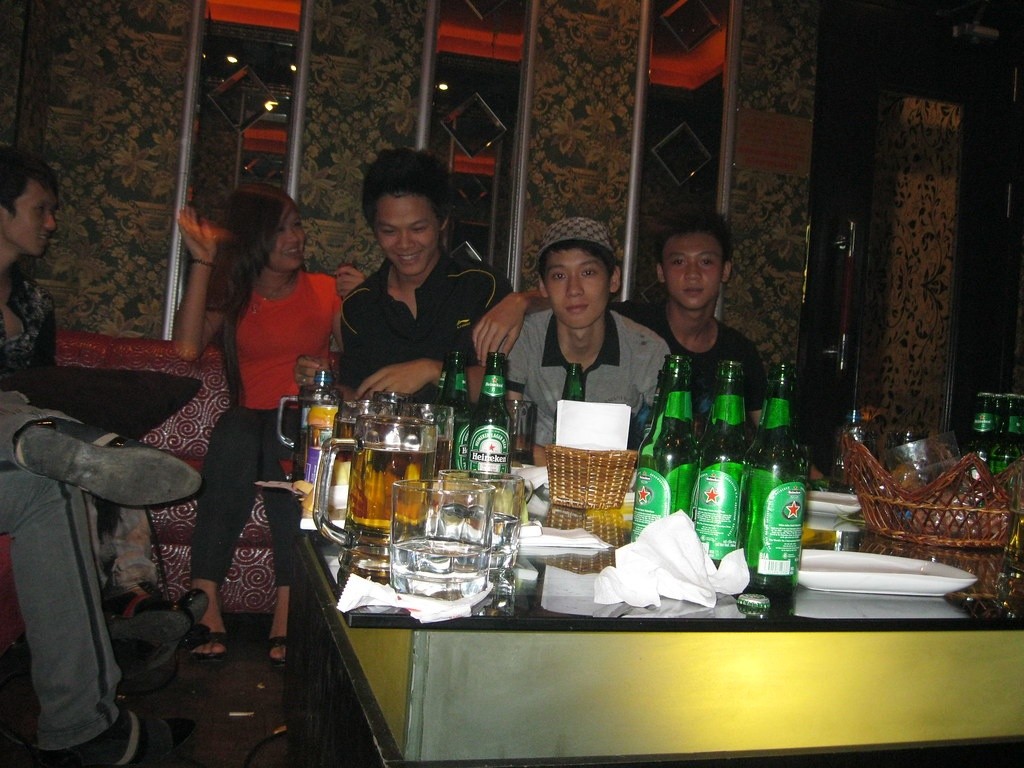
[294,149,514,404]
[174,181,365,663]
[0,390,202,768]
[473,205,822,479]
[498,216,670,465]
[0,149,211,688]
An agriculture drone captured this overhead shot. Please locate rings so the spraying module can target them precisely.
[303,376,307,382]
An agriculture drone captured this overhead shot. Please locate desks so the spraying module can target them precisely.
[265,461,1024,768]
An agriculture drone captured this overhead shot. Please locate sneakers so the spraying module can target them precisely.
[137,588,208,657]
[104,592,192,641]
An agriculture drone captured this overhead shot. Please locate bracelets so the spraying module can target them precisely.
[189,259,215,267]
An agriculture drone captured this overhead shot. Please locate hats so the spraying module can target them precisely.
[535,217,612,261]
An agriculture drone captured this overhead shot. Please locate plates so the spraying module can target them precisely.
[805,516,860,532]
[798,548,978,596]
[793,589,968,619]
[807,490,861,517]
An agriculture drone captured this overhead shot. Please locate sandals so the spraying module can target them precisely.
[192,632,228,661]
[268,636,287,663]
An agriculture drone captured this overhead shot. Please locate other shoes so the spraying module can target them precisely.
[14,417,202,507]
[37,709,196,768]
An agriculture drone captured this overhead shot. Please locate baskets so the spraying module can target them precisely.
[545,443,637,507]
[541,507,626,575]
[858,536,1005,618]
[840,434,1024,547]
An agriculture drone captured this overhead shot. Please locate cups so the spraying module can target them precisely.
[505,400,538,467]
[390,479,496,600]
[331,393,455,471]
[1005,460,1024,573]
[440,469,525,596]
[276,384,342,481]
[313,415,439,555]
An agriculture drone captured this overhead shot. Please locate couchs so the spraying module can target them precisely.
[0,327,341,665]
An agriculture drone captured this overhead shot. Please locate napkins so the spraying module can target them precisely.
[595,509,751,607]
[512,465,549,490]
[333,573,472,623]
[516,522,613,549]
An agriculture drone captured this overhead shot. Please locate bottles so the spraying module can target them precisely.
[312,369,332,386]
[432,351,470,469]
[551,363,585,445]
[629,355,807,597]
[830,406,876,494]
[966,392,1024,474]
[470,352,511,473]
[305,405,338,483]
